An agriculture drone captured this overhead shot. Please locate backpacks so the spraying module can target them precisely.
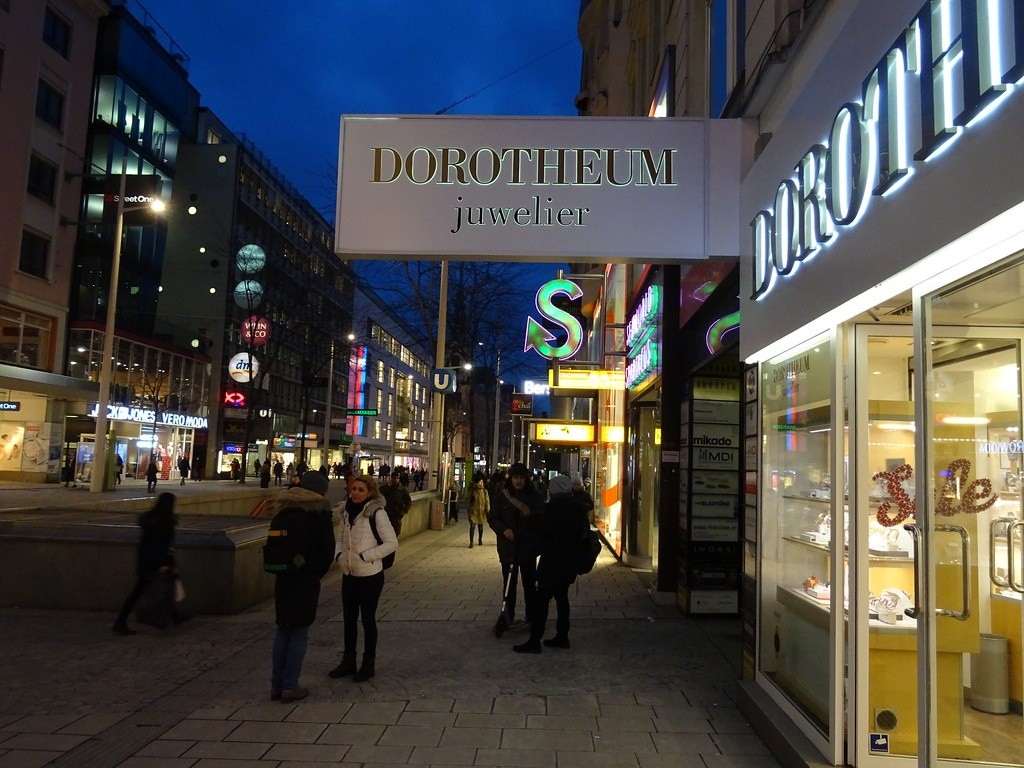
[369,508,399,570]
[263,507,312,575]
[576,531,601,573]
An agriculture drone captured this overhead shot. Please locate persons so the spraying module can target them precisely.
[486,463,546,639]
[452,481,461,493]
[379,471,412,538]
[467,477,490,548]
[147,459,161,493]
[513,475,594,654]
[473,469,594,515]
[116,454,123,485]
[230,458,347,489]
[368,463,425,491]
[111,491,181,635]
[328,475,399,683]
[179,457,191,486]
[193,457,204,481]
[263,470,336,703]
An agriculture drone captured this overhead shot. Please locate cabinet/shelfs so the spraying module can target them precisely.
[781,476,918,621]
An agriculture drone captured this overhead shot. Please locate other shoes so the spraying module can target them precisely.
[111,621,136,635]
[479,539,482,545]
[271,686,309,703]
[469,542,473,548]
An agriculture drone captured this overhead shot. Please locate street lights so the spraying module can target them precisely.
[312,333,357,477]
[427,260,474,490]
[90,155,168,493]
[479,341,504,475]
[508,416,537,469]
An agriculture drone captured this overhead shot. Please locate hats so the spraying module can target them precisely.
[303,471,328,490]
[508,463,530,477]
[550,476,572,493]
[391,472,400,476]
[572,476,583,487]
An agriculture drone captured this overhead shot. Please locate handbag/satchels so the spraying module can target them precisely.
[148,565,184,601]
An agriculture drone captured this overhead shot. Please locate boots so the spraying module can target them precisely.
[544,620,573,648]
[328,650,357,678]
[513,621,546,653]
[352,652,375,682]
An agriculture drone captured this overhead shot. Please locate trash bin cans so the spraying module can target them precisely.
[429,500,445,531]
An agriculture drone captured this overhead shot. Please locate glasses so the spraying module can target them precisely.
[356,474,367,482]
